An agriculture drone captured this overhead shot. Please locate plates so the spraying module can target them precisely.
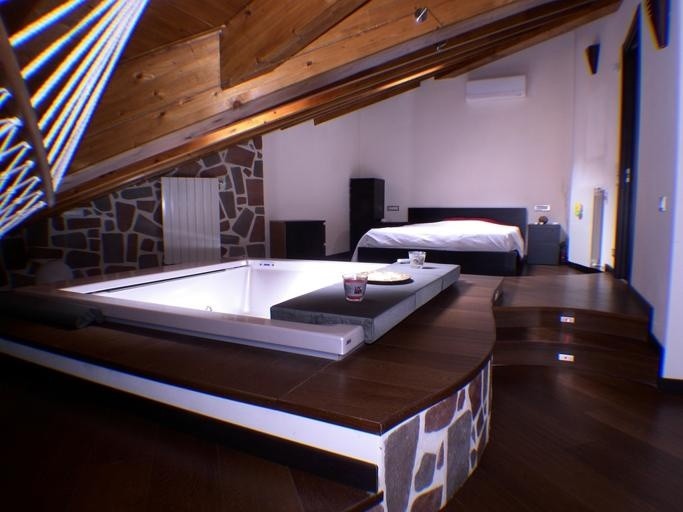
[366,271,411,283]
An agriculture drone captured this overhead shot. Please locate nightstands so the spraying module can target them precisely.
[525,223,562,266]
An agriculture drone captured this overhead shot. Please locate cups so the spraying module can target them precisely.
[409,251,426,269]
[343,272,368,302]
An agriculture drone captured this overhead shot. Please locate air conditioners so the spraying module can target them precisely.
[464,74,526,103]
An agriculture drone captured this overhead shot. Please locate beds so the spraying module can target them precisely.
[356,206,528,276]
